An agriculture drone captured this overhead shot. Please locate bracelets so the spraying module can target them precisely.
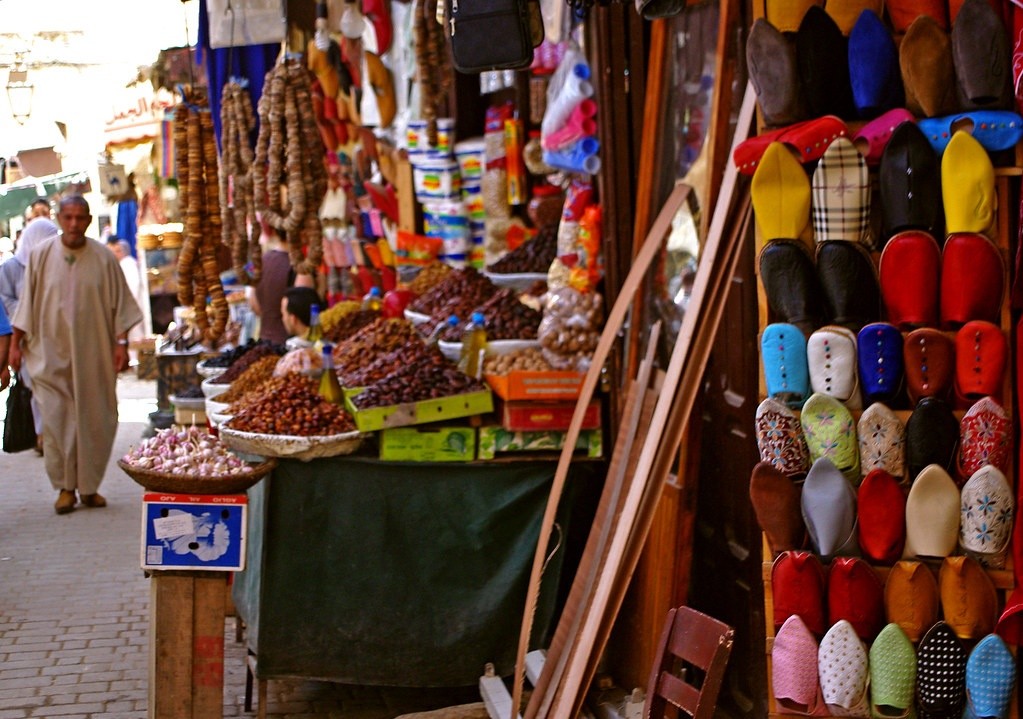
[118,339,128,345]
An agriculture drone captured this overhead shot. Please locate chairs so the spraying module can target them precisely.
[642,607,735,719]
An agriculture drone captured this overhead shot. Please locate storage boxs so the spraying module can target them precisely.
[140,490,248,571]
[149,571,227,719]
[338,368,603,461]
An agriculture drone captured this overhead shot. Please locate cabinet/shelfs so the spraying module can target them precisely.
[752,0,1022,719]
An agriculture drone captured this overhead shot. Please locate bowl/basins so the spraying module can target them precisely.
[438,339,540,362]
[402,310,432,331]
[198,359,239,424]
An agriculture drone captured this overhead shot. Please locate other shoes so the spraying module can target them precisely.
[54,488,77,514]
[741,1,1022,718]
[80,493,106,507]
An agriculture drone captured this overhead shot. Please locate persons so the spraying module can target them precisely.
[0,299,14,374]
[280,286,322,336]
[251,229,297,346]
[30,199,52,220]
[0,218,58,453]
[107,235,141,369]
[8,196,143,514]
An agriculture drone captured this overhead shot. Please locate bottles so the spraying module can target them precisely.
[460,312,485,380]
[359,287,382,331]
[423,316,458,350]
[308,303,323,344]
[319,342,344,410]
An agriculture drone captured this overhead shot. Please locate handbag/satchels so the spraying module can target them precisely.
[3,372,37,454]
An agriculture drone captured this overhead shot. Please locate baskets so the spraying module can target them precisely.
[117,459,279,494]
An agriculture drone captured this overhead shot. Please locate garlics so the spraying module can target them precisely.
[122,412,254,477]
